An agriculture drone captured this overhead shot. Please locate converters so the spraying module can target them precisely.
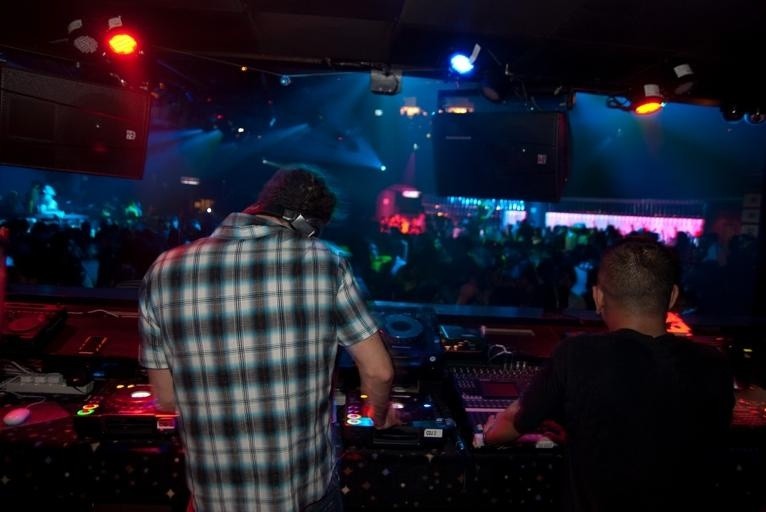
[67,372,91,387]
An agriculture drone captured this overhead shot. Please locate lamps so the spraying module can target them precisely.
[478,69,512,104]
[720,89,766,124]
[371,65,398,94]
[619,64,670,116]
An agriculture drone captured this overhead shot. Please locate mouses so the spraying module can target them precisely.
[3,409,29,426]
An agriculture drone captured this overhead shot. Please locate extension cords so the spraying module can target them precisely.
[6,372,96,395]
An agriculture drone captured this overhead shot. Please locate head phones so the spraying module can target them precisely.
[242,203,323,237]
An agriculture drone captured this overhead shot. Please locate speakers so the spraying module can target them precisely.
[0,64,153,181]
[432,111,572,203]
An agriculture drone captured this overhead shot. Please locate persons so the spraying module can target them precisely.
[356,212,764,321]
[1,213,212,288]
[137,167,395,510]
[485,241,736,510]
